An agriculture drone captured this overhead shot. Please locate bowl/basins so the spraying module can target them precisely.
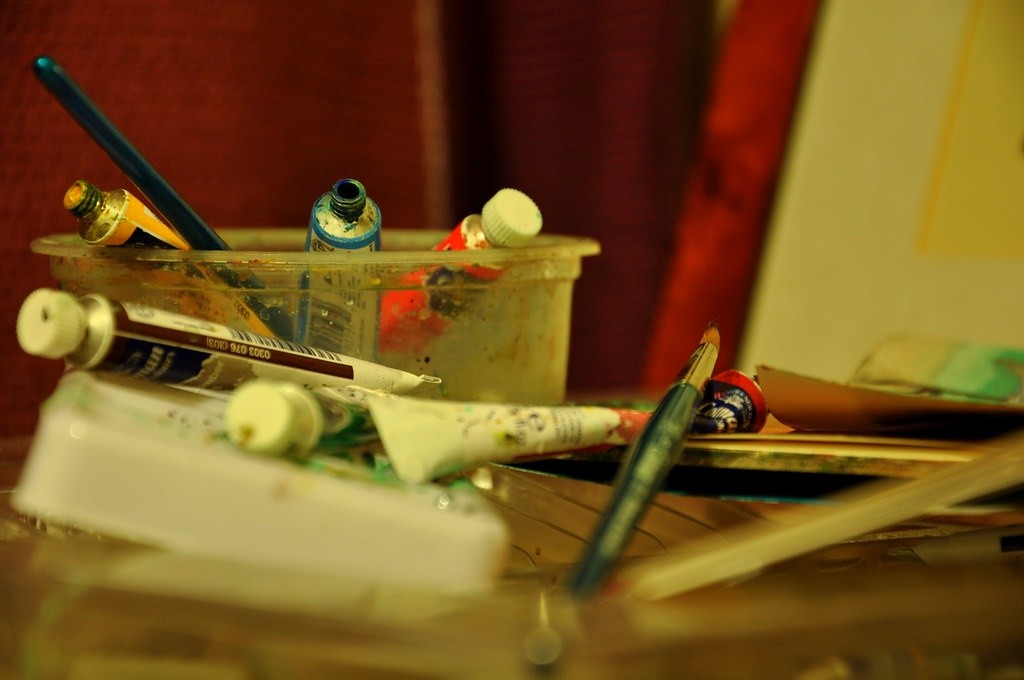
[31,230,603,411]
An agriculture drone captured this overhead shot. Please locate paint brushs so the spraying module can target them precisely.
[566,319,724,606]
